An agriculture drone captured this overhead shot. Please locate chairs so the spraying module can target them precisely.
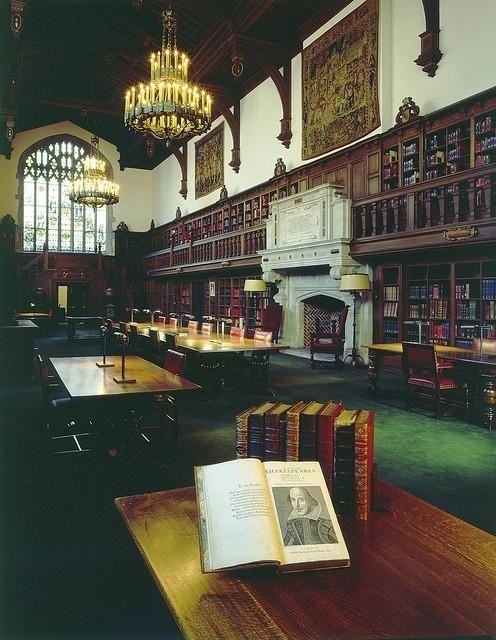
[107,319,112,334]
[33,349,186,454]
[402,342,469,420]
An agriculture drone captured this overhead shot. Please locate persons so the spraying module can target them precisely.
[280,485,339,545]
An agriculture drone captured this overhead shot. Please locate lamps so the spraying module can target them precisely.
[124,0,212,149]
[70,137,119,211]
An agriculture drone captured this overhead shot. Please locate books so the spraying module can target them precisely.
[382,278,496,348]
[380,113,496,205]
[234,400,376,521]
[182,279,277,339]
[180,186,298,242]
[194,459,354,575]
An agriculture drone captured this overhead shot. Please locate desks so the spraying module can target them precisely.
[113,476,496,640]
[66,315,105,340]
[361,344,496,428]
